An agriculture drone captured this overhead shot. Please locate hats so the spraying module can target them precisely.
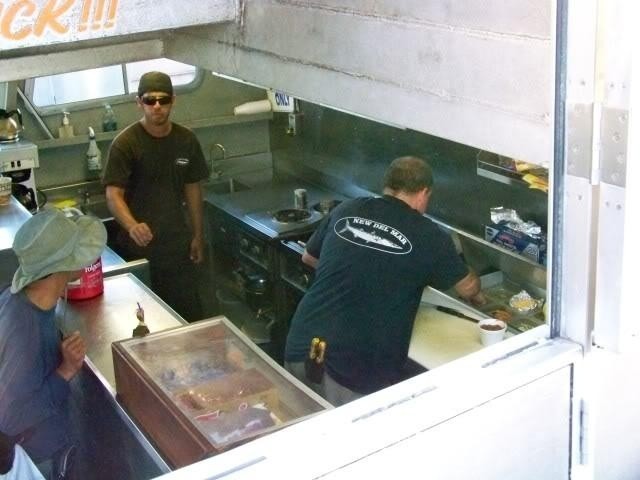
[8,206,109,295]
[137,71,174,96]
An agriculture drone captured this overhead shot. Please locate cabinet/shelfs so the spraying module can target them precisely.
[278,249,317,349]
[204,200,277,358]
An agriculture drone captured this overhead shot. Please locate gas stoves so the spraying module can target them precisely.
[246,198,348,240]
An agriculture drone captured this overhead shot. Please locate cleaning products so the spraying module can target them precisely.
[59,111,75,140]
[85,126,102,171]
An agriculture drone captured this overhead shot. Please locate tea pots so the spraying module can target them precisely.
[12,184,38,215]
[0,108,27,143]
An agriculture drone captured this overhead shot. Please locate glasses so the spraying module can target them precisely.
[142,94,171,106]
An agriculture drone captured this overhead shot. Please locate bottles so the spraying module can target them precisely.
[131,301,150,337]
[294,189,307,209]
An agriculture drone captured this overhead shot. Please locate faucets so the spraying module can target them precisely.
[207,143,226,178]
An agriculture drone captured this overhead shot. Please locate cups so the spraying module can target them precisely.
[478,320,507,346]
[1,177,12,206]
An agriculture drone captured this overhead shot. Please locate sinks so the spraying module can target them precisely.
[203,177,250,197]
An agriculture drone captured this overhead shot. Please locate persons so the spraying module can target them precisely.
[283,157,480,406]
[106,73,211,323]
[0,209,107,480]
